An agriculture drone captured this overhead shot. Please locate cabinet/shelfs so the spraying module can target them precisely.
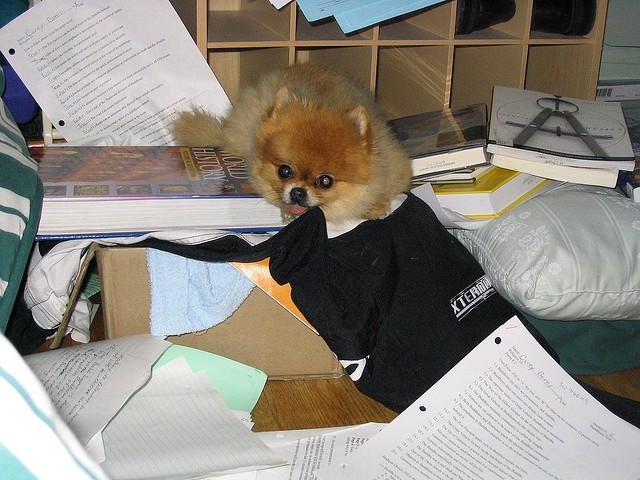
[167,1,609,122]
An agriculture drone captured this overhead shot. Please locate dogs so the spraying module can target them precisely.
[170,61,412,224]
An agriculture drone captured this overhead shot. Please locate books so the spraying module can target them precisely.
[35,146,307,241]
[409,167,552,219]
[385,103,498,184]
[486,85,640,202]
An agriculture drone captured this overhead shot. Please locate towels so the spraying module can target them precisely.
[146,247,255,337]
